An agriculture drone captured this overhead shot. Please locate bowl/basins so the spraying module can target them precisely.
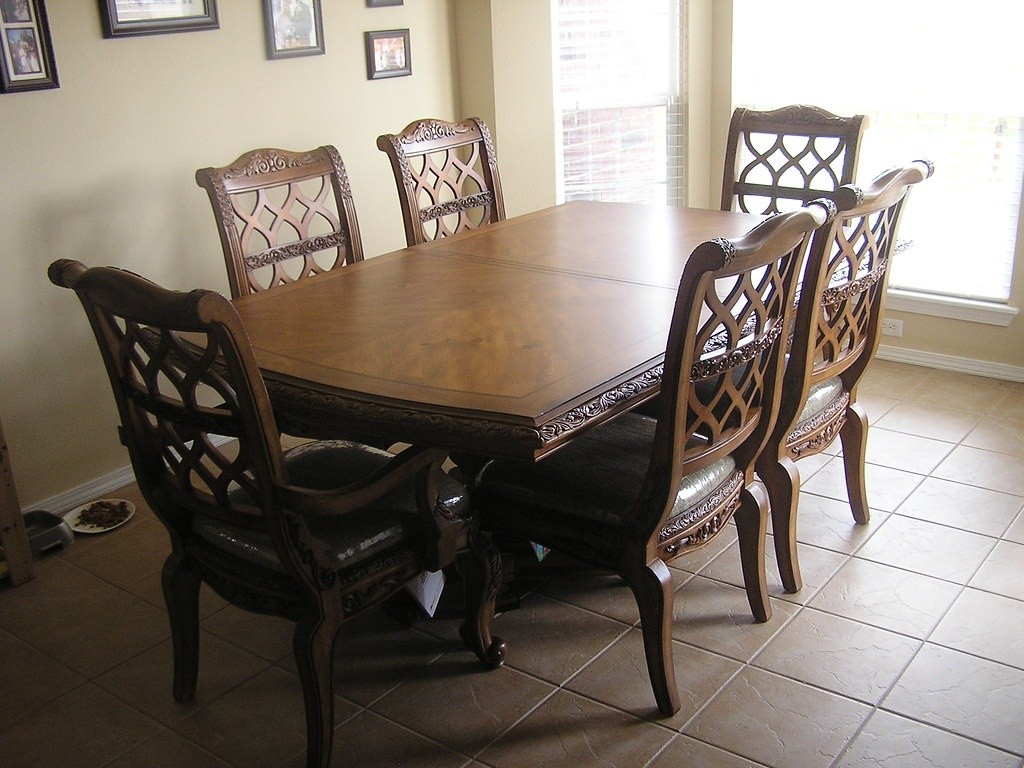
[22,509,75,562]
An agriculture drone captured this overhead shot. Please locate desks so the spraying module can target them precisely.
[182,199,915,611]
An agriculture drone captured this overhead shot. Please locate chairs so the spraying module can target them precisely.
[720,103,869,227]
[630,156,936,593]
[457,197,836,716]
[48,259,509,768]
[196,145,365,296]
[377,115,506,247]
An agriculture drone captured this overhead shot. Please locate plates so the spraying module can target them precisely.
[62,499,136,534]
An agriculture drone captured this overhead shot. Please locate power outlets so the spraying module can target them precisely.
[880,316,906,339]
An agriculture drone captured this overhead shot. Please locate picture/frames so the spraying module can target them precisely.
[365,27,413,81]
[0,0,61,94]
[262,0,326,60]
[367,0,404,7]
[100,0,219,37]
